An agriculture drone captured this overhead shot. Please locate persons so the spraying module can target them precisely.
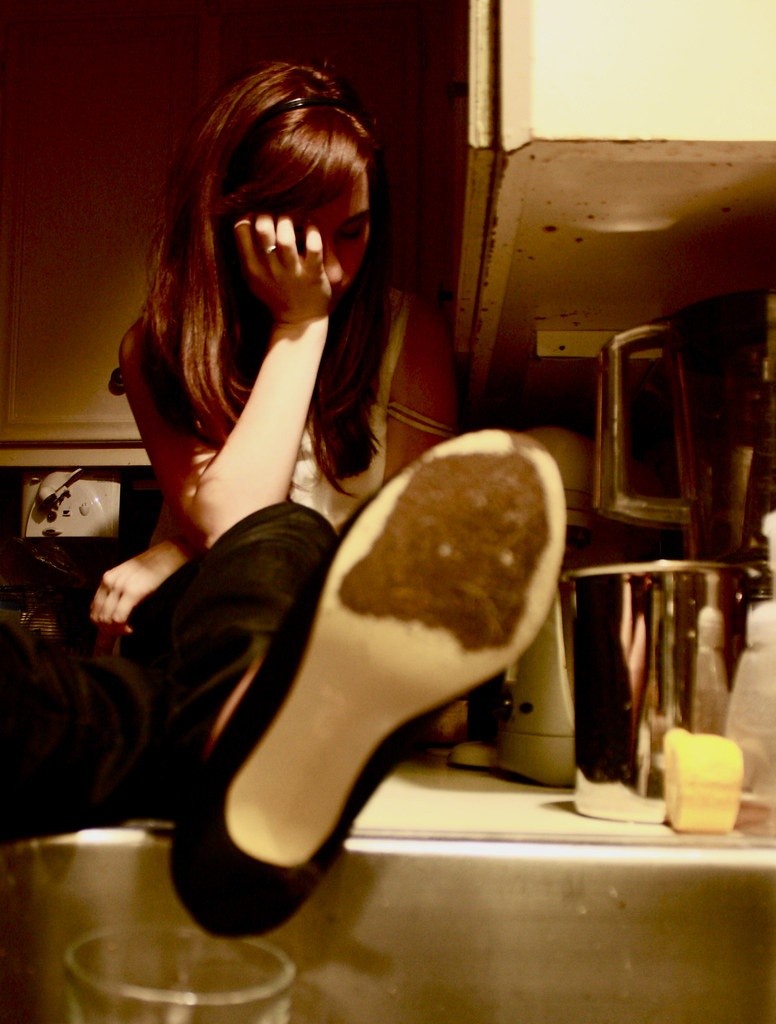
[0,61,566,939]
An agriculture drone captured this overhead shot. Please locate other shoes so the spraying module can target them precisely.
[168,430,571,943]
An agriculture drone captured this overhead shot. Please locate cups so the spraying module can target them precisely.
[60,921,302,1024]
[594,287,776,625]
[565,562,732,825]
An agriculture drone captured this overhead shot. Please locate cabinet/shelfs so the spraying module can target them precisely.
[0,0,776,469]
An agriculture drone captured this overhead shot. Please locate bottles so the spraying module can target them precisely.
[727,598,776,838]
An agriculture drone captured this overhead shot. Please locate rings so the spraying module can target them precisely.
[264,246,276,254]
[234,220,250,229]
